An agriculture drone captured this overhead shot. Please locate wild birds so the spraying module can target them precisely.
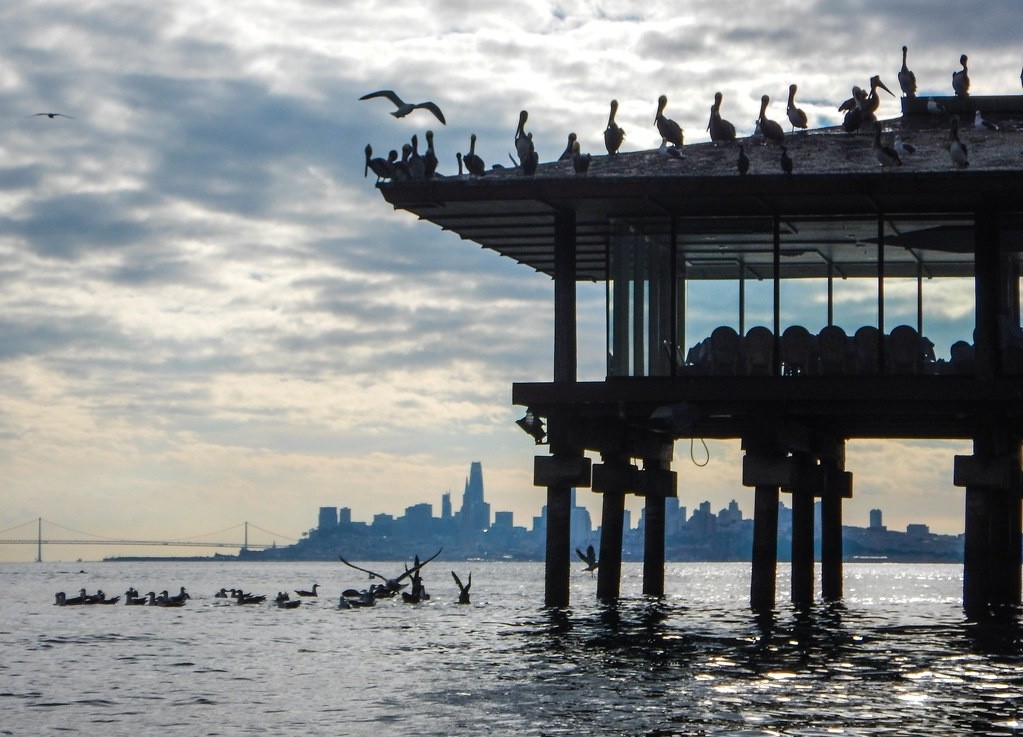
[576,545,598,579]
[514,111,538,178]
[358,90,446,124]
[456,133,486,179]
[754,95,784,144]
[706,92,736,140]
[737,146,749,174]
[952,55,970,95]
[898,46,916,98]
[334,547,473,609]
[364,130,438,182]
[55,587,190,608]
[558,133,590,175]
[604,100,625,158]
[653,95,684,159]
[838,75,998,168]
[780,147,792,174]
[216,584,320,608]
[786,85,806,133]
[29,112,72,119]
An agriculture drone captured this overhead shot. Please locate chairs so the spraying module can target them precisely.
[699,324,979,378]
